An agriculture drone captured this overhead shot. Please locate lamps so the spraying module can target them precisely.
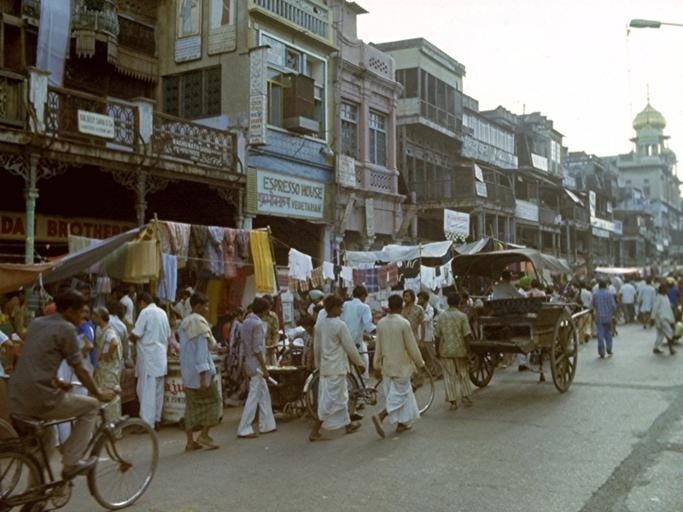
[293,130,338,159]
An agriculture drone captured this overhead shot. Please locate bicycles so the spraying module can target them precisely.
[0,379,160,511]
[303,336,435,424]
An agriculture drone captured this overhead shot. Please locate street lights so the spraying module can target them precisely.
[628,19,681,29]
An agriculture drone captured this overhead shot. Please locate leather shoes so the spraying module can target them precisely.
[61,455,97,480]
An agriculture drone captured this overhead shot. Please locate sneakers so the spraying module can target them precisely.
[396,425,412,432]
[351,413,362,420]
[373,414,386,437]
[195,433,219,449]
[184,441,203,451]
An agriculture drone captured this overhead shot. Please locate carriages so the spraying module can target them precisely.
[451,250,593,393]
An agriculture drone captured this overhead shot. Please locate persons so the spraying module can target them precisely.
[590,261,682,347]
[434,292,473,411]
[128,292,171,434]
[92,306,122,441]
[591,279,618,358]
[648,284,676,354]
[2,285,281,408]
[309,294,366,441]
[488,268,597,371]
[301,285,383,417]
[402,288,425,344]
[458,290,478,340]
[55,330,94,461]
[372,294,426,439]
[6,287,115,512]
[236,298,277,438]
[617,278,636,324]
[177,291,224,450]
[416,291,443,386]
